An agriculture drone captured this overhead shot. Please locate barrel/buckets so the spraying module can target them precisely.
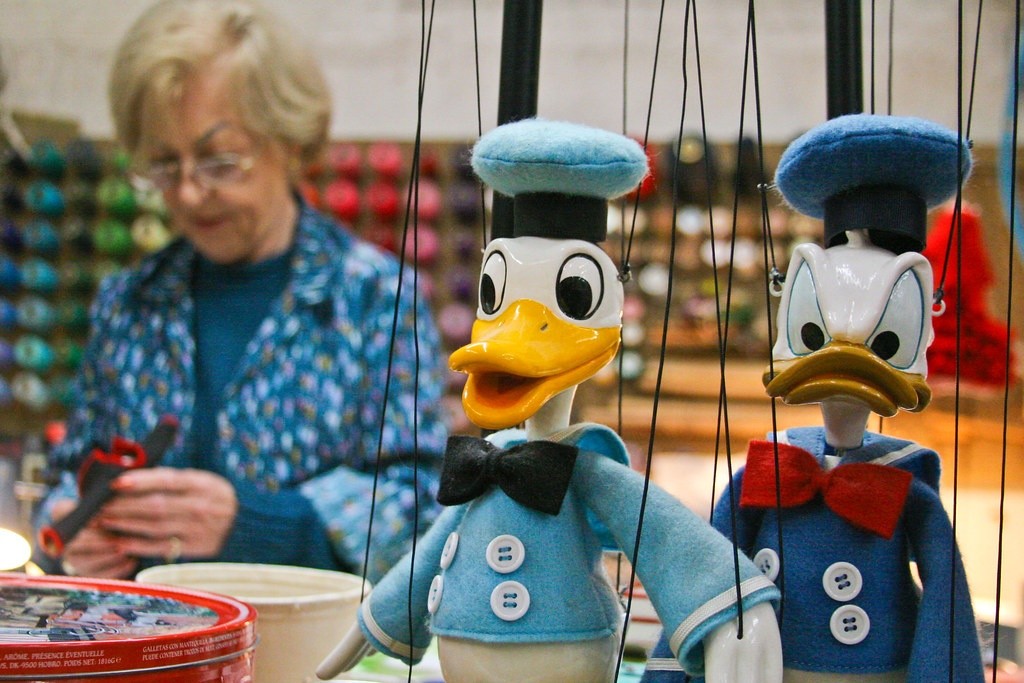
[1,572,259,683]
[132,561,370,683]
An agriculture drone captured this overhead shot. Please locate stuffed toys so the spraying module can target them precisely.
[715,109,996,683]
[315,116,787,683]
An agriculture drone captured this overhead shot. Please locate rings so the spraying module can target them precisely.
[165,534,180,562]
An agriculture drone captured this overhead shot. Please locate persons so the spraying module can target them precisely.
[22,0,448,586]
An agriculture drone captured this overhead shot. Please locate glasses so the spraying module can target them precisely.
[135,145,258,192]
